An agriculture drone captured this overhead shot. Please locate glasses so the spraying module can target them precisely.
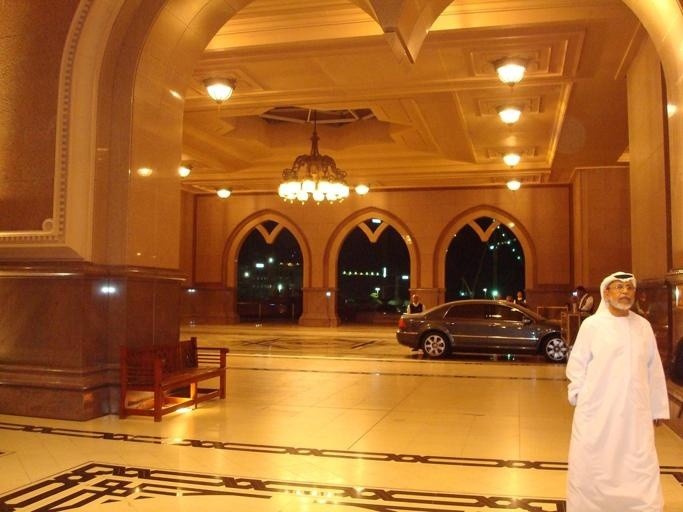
[608,284,636,291]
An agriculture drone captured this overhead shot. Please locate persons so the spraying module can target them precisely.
[570,285,594,314]
[563,269,671,512]
[505,295,512,302]
[512,290,526,306]
[405,294,425,313]
[564,302,571,311]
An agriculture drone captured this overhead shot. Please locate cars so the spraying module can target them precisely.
[395,299,568,363]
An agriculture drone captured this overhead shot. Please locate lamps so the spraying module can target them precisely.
[175,160,195,178]
[274,109,354,207]
[200,75,236,105]
[211,185,235,202]
[353,181,371,198]
[489,52,530,193]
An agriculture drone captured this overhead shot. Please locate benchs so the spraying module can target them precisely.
[114,334,229,424]
